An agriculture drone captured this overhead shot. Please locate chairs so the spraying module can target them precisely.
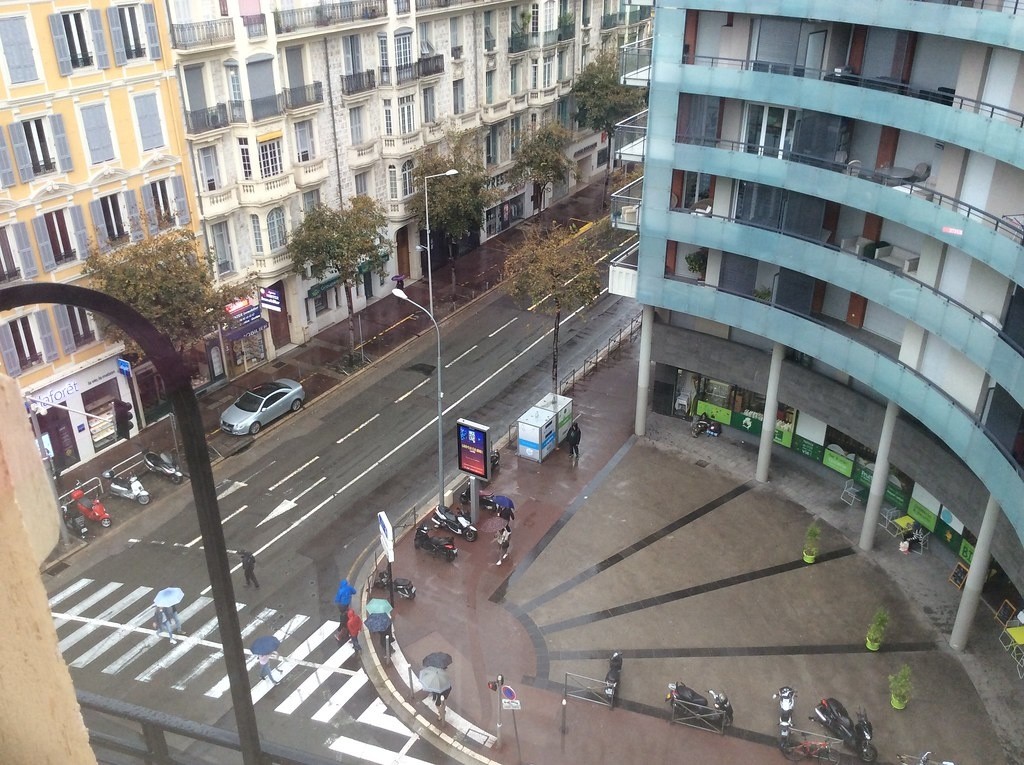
[840,479,861,506]
[842,160,862,177]
[1017,654,1024,680]
[885,510,903,537]
[621,205,637,223]
[903,162,929,184]
[912,531,930,555]
[999,620,1020,651]
[878,506,897,529]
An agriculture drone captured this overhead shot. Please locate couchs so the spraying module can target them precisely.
[840,236,875,256]
[919,86,955,105]
[875,245,920,272]
[823,73,859,86]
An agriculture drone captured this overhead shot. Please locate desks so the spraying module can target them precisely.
[1007,627,1024,655]
[874,166,914,187]
[855,487,870,504]
[893,515,915,539]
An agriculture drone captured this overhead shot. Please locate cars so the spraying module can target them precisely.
[219,378,306,436]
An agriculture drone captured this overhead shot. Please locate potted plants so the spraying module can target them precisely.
[888,663,914,711]
[802,520,823,563]
[315,6,324,25]
[865,605,891,651]
[273,7,283,34]
[684,250,708,286]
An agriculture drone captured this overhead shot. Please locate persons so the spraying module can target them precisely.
[692,412,711,438]
[335,580,357,631]
[499,507,515,526]
[377,630,396,659]
[257,654,280,685]
[492,528,505,568]
[566,421,581,460]
[236,547,260,589]
[153,608,177,645]
[334,604,352,642]
[435,686,452,721]
[156,606,187,635]
[348,608,363,649]
[503,523,512,554]
[906,528,925,549]
[396,280,404,291]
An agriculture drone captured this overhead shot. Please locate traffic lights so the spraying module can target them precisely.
[114,398,135,440]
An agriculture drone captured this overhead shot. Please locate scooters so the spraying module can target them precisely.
[772,686,798,749]
[430,503,478,542]
[414,523,460,563]
[60,501,89,541]
[809,697,878,764]
[102,468,151,506]
[663,681,733,729]
[71,488,112,529]
[143,448,183,484]
[374,564,417,600]
[603,669,622,706]
[459,481,498,512]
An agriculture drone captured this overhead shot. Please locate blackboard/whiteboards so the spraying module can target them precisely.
[949,562,969,589]
[995,600,1016,626]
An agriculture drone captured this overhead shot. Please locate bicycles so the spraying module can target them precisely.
[897,750,955,765]
[781,731,842,765]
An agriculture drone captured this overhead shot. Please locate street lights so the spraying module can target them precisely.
[415,169,459,318]
[393,288,445,507]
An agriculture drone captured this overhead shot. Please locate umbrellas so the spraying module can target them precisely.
[391,274,405,281]
[423,652,453,668]
[365,597,393,616]
[495,495,515,508]
[250,635,282,655]
[153,586,185,609]
[418,666,451,693]
[364,613,393,633]
